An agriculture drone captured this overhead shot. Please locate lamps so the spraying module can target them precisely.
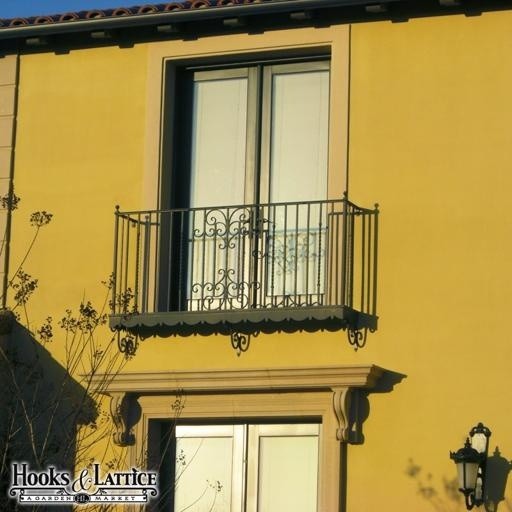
[446,420,492,511]
